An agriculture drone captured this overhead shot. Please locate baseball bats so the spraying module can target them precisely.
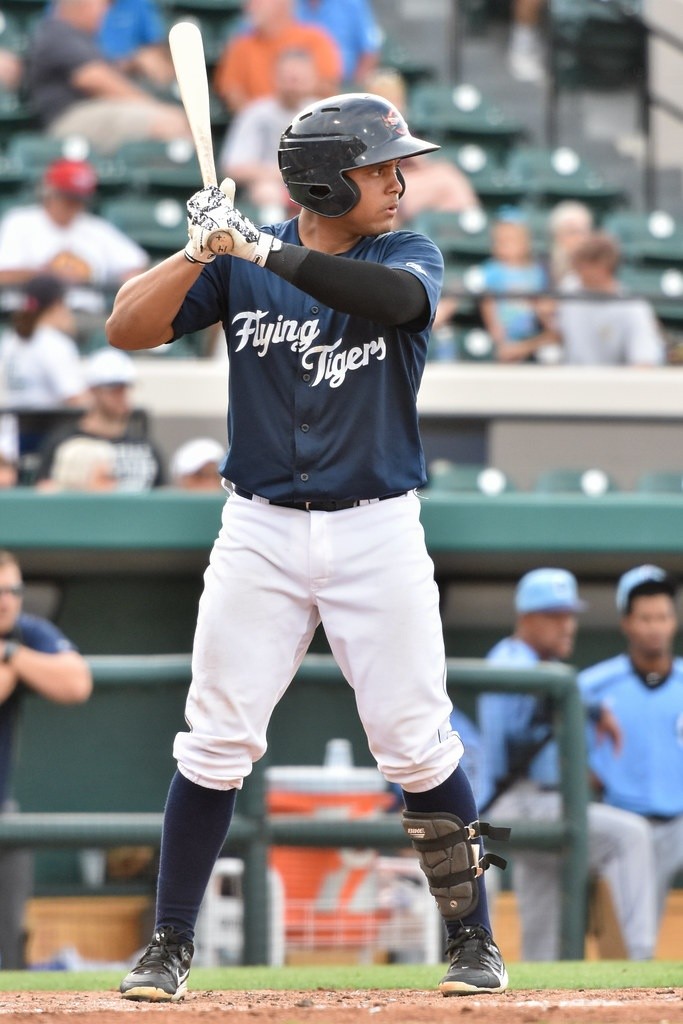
[169,21,218,190]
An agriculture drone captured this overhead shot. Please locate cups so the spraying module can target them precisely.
[324,738,355,768]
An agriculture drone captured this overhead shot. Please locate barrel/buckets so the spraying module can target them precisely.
[264,766,397,966]
[22,895,152,968]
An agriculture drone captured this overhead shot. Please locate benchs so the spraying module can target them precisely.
[0,0,683,493]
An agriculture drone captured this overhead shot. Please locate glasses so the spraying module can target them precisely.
[0,584,25,598]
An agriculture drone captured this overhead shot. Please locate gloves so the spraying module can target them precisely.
[185,178,284,269]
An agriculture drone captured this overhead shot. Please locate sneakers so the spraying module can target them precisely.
[440,922,508,996]
[120,925,196,998]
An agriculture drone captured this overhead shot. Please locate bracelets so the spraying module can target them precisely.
[2,639,17,663]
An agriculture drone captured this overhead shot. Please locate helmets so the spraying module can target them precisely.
[278,93,442,218]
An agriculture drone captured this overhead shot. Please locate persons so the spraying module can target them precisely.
[0,0,683,1000]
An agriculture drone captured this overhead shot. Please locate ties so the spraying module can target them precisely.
[231,483,406,512]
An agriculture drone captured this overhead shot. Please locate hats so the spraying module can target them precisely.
[610,562,674,613]
[81,346,135,388]
[169,435,227,477]
[44,158,99,199]
[508,567,587,618]
